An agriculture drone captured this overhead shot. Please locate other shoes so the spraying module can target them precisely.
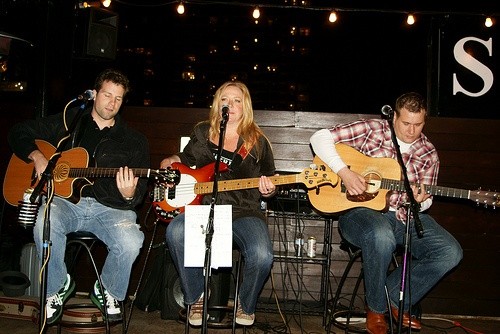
[188,297,203,326]
[235,294,255,325]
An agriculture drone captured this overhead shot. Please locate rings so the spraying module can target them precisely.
[267,189,269,191]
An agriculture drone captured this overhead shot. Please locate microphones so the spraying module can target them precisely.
[221,103,229,124]
[381,105,394,116]
[78,89,94,101]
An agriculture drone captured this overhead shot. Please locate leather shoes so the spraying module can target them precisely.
[391,306,422,330]
[365,309,387,334]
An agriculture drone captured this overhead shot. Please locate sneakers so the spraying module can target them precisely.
[90,280,123,321]
[45,274,75,325]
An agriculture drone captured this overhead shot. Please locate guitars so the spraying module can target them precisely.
[152,161,339,224]
[306,142,500,217]
[3,139,181,208]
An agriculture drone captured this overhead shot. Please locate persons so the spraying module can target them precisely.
[160,82,279,325]
[309,93,463,334]
[0,71,153,323]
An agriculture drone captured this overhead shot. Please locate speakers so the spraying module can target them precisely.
[76,7,120,58]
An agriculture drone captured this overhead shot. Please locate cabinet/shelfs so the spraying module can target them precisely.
[256,210,333,326]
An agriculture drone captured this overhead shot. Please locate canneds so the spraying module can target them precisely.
[307,237,316,258]
[295,238,305,256]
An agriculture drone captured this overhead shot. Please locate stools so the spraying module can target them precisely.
[57,233,127,334]
[327,245,409,334]
[185,251,246,334]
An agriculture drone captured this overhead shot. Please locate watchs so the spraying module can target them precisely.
[125,197,133,201]
